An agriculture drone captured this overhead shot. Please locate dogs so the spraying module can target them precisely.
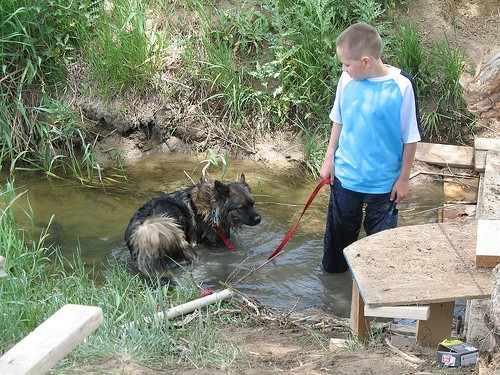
[124,173,263,279]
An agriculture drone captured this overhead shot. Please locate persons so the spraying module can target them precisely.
[319,22,422,273]
